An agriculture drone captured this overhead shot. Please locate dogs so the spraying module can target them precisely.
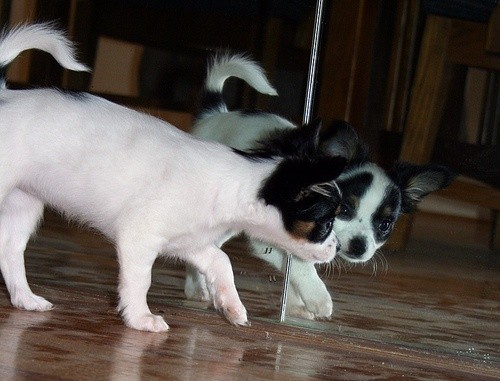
[0,20,348,335]
[189,50,457,323]
